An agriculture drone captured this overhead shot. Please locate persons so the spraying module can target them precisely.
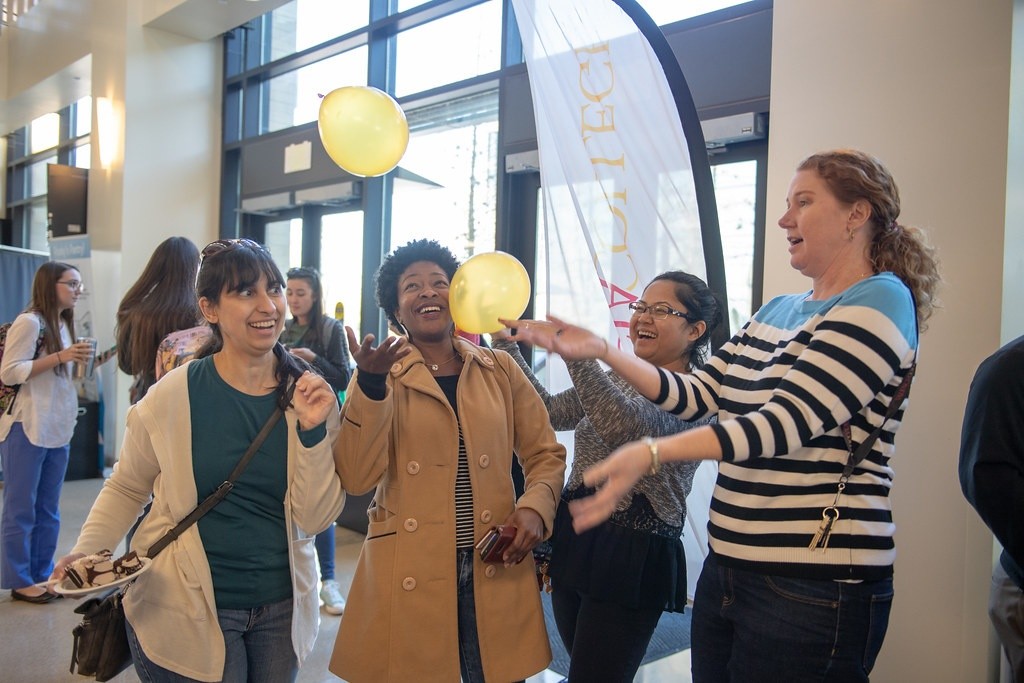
[115,236,213,555]
[46,238,346,683]
[1,262,95,601]
[330,237,568,683]
[279,267,351,615]
[491,271,722,682]
[958,333,1024,683]
[502,152,939,683]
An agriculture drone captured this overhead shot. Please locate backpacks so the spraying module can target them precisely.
[0,314,46,416]
[154,326,216,381]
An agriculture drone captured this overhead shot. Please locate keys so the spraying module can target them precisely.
[809,506,840,554]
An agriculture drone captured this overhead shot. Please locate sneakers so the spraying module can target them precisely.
[322,578,346,615]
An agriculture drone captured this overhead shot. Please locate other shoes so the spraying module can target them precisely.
[11,588,53,604]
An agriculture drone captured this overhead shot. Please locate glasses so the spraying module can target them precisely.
[200,238,262,271]
[628,301,693,321]
[57,280,84,292]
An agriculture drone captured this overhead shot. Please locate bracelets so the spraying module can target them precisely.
[601,338,608,360]
[57,351,63,364]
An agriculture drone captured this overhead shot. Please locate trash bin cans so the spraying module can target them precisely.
[63,400,100,482]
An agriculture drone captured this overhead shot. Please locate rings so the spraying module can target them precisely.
[526,322,528,328]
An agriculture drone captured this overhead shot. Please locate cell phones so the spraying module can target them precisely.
[97,345,118,360]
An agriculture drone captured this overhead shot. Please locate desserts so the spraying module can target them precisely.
[61,549,144,589]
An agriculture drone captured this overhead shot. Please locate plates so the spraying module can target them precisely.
[54,557,153,596]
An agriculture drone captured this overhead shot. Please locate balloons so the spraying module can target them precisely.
[317,86,410,177]
[448,251,531,337]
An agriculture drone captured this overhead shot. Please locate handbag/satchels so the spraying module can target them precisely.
[69,595,132,683]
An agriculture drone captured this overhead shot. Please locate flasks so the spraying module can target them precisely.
[72,337,97,378]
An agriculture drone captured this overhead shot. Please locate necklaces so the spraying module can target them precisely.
[426,353,459,371]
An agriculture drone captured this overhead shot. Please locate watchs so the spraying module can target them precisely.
[641,435,662,476]
